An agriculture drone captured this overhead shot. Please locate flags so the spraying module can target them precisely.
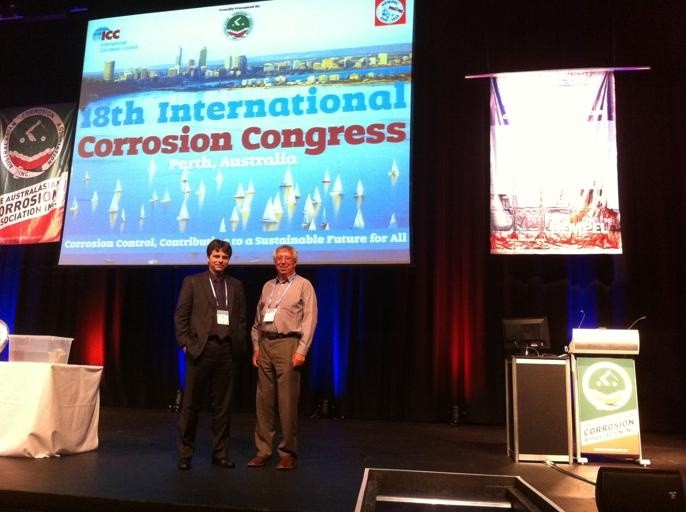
[485,72,623,256]
[0,101,77,244]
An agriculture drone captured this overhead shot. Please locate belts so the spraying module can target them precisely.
[262,331,301,340]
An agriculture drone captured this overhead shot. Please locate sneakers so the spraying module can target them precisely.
[276,456,297,469]
[248,457,272,468]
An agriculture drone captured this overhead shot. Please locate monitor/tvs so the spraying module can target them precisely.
[502,318,551,359]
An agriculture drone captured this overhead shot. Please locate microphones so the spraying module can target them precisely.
[578,309,586,327]
[628,316,647,329]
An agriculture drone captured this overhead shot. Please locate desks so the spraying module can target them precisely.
[0,360,103,457]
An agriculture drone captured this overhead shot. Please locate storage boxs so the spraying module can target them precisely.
[6,334,74,364]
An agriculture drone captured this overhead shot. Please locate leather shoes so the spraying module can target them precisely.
[222,460,235,468]
[177,456,192,470]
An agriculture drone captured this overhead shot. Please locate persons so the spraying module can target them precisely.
[172,239,247,469]
[249,244,317,470]
